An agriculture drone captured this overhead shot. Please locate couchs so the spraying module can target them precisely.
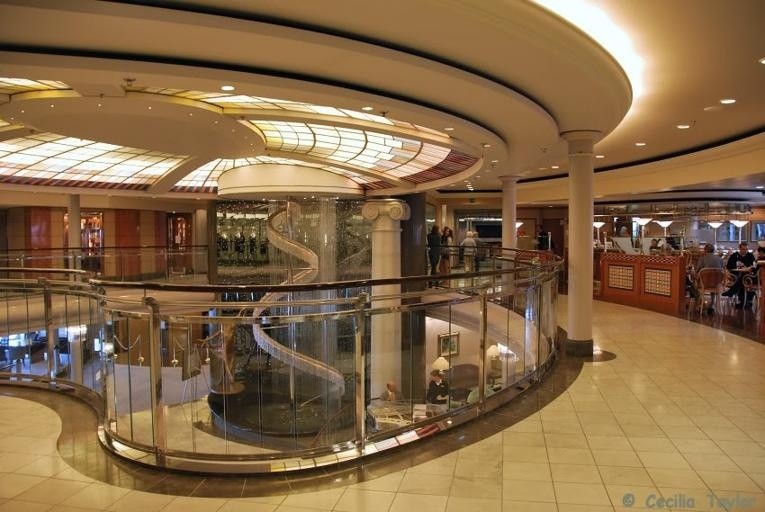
[444,364,479,391]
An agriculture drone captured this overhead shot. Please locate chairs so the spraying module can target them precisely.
[682,249,765,316]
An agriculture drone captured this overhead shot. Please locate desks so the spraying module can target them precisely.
[488,371,502,385]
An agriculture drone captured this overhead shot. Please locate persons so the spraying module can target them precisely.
[460,231,475,275]
[439,226,455,285]
[721,247,765,297]
[426,224,441,288]
[471,232,483,272]
[531,224,554,250]
[727,242,754,309]
[691,243,722,314]
[381,382,404,401]
[427,369,449,404]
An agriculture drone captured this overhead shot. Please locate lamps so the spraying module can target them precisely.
[636,218,651,238]
[487,345,499,371]
[656,221,673,236]
[708,222,723,243]
[730,220,748,243]
[432,357,449,380]
[593,221,605,239]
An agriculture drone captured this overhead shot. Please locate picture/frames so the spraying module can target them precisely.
[438,331,460,359]
